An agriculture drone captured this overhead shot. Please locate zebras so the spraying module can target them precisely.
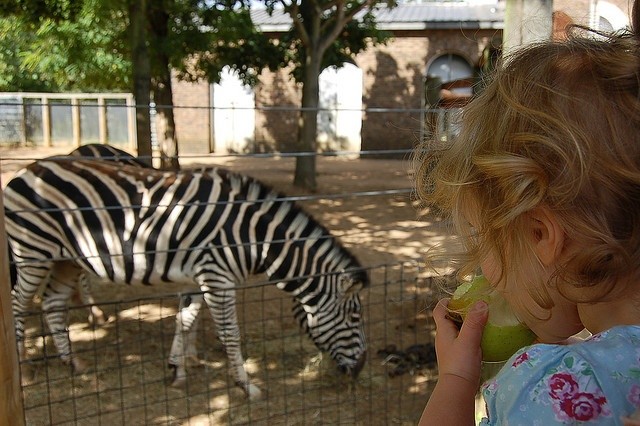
[35,144,159,325]
[3,155,370,400]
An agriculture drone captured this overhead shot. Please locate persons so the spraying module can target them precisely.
[400,19,636,425]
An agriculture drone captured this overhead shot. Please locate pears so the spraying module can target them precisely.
[447,276,537,378]
